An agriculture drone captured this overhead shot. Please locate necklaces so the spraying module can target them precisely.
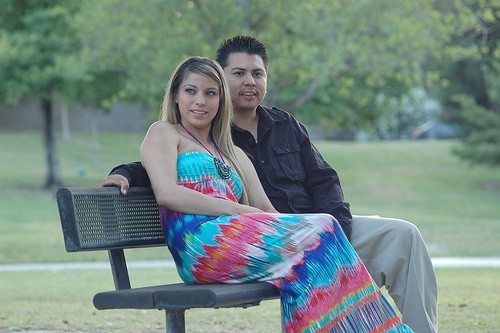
[180,119,232,179]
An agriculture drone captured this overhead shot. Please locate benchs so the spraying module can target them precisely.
[56,185,285,333]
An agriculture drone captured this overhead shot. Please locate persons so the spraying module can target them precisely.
[97,36,438,332]
[142,57,415,333]
